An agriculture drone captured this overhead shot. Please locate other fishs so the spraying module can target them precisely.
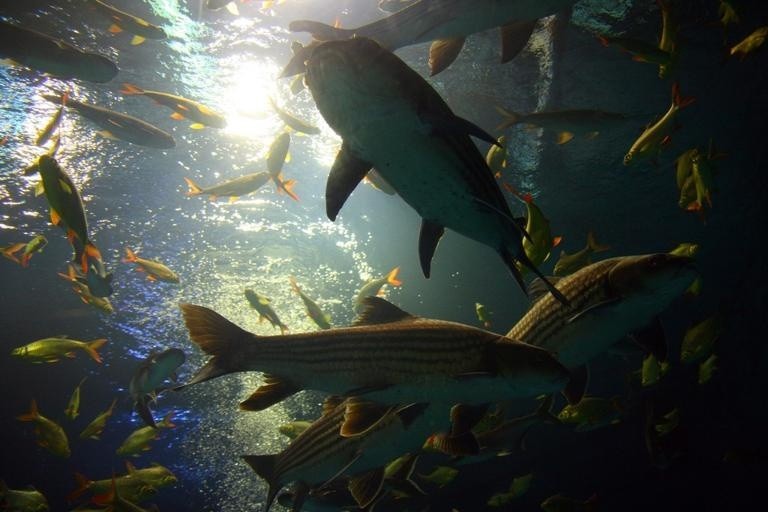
[0,0,721,512]
